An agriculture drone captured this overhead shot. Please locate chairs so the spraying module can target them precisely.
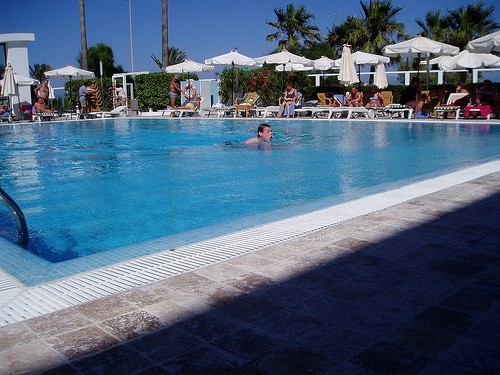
[32,106,53,122]
[89,106,126,118]
[210,92,414,120]
[158,97,205,117]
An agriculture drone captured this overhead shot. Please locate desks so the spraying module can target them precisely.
[465,104,491,121]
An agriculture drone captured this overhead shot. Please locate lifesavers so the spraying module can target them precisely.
[184,84,196,98]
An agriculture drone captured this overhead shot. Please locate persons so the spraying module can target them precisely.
[244,124,273,146]
[0,74,500,120]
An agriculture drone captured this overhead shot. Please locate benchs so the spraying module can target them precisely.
[434,92,470,120]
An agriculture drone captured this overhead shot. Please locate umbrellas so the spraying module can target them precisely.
[0,63,41,97]
[44,65,96,81]
[204,50,264,67]
[419,49,500,70]
[166,57,215,75]
[466,31,500,52]
[253,49,390,72]
[381,36,459,56]
